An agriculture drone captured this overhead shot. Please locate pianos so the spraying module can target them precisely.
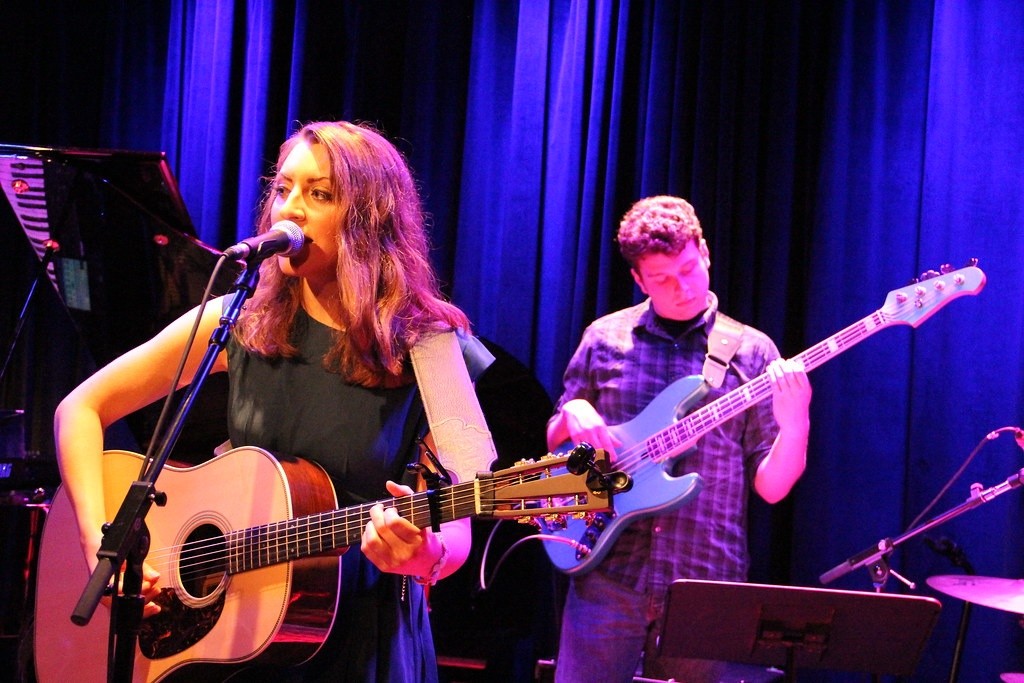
[0,143,559,683]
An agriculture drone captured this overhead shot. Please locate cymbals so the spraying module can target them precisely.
[925,574,1024,616]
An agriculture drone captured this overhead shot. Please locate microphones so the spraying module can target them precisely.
[223,220,305,260]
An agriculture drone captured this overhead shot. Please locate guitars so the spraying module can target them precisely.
[536,255,988,577]
[32,443,631,683]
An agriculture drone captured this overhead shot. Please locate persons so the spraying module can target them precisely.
[546,195,811,683]
[54,119,474,683]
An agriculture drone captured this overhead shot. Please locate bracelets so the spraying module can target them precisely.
[411,537,449,585]
